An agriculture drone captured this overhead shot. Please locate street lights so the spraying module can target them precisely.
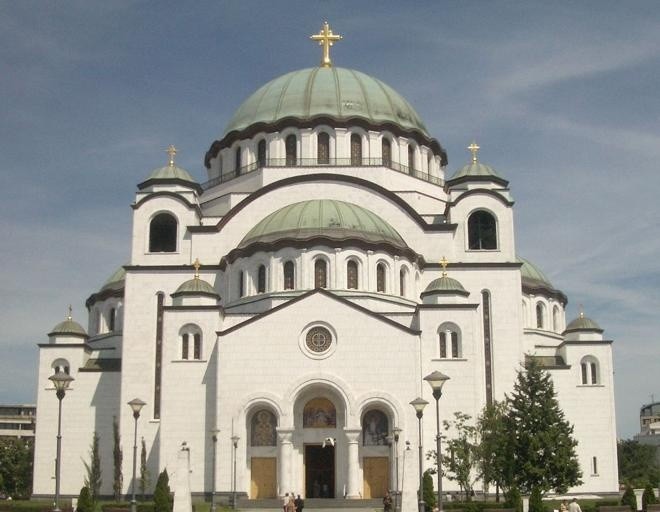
[390,424,403,511]
[409,396,429,511]
[48,370,74,511]
[208,427,221,512]
[127,397,146,511]
[423,368,449,511]
[230,435,240,510]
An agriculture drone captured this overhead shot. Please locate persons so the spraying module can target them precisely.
[432,487,478,512]
[567,498,582,512]
[281,493,305,512]
[560,500,568,512]
[383,492,392,512]
